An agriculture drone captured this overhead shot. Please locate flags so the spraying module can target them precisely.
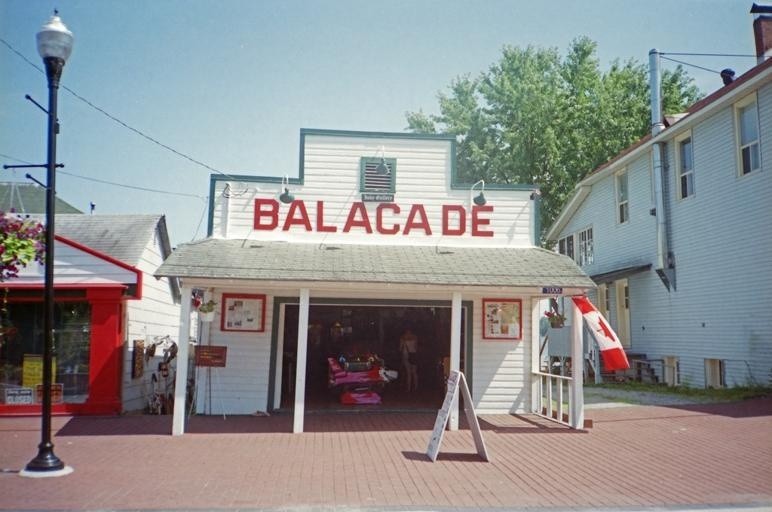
[571,295,631,371]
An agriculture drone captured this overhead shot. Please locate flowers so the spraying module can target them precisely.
[0,208,45,281]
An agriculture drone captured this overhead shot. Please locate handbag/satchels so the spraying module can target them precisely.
[408,352,419,362]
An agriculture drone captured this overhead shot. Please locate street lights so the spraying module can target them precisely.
[25,9,75,472]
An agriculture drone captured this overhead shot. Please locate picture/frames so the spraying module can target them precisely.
[483,299,522,340]
[220,293,266,332]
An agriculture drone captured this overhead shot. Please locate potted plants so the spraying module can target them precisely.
[198,301,216,322]
[545,310,566,328]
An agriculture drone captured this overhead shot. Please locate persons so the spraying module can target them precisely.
[397,320,420,394]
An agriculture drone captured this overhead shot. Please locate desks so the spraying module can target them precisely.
[327,352,398,402]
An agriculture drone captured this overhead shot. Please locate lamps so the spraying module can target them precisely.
[280,174,294,204]
[470,181,486,205]
[376,145,389,175]
[331,311,344,329]
